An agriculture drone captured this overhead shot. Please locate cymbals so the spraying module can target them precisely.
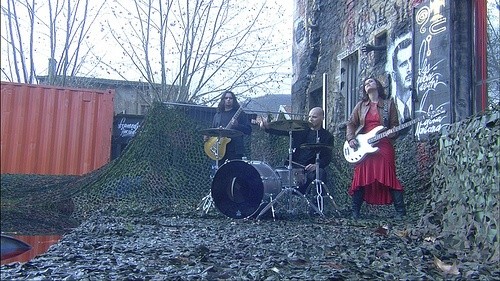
[262,120,313,136]
[300,142,334,150]
[198,127,243,138]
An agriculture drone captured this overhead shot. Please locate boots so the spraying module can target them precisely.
[391,189,406,220]
[349,187,364,220]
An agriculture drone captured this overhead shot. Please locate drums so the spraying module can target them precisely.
[211,157,280,220]
[272,167,307,188]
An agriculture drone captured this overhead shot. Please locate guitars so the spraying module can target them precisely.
[203,97,253,160]
[342,107,447,163]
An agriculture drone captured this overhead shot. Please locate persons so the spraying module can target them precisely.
[203,91,252,165]
[284,108,334,215]
[346,78,407,220]
